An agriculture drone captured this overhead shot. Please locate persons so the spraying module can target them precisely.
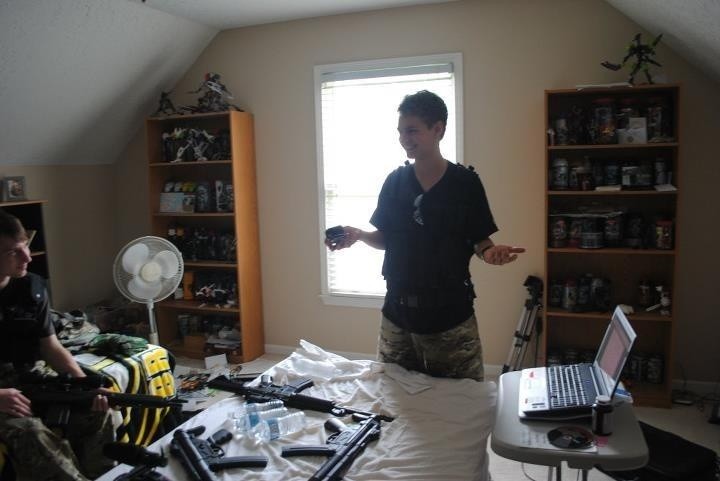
[0,210,117,481]
[324,90,526,382]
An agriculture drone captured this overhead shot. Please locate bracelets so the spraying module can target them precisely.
[480,244,495,261]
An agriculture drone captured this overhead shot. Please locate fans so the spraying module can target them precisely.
[113,235,185,347]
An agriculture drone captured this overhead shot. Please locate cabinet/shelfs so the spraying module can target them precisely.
[542,84,683,411]
[0,199,52,309]
[142,111,267,365]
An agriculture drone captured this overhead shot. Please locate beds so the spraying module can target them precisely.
[1,334,177,481]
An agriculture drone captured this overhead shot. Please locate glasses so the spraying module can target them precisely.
[411,192,425,226]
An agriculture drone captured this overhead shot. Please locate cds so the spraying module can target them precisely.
[548,426,594,449]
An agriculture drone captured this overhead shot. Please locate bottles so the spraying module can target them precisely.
[547,119,593,191]
[591,396,614,436]
[549,277,603,312]
[653,219,674,250]
[230,400,308,442]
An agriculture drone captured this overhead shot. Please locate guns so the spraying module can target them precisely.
[170,426,267,480]
[19,374,189,425]
[281,411,380,480]
[208,375,395,423]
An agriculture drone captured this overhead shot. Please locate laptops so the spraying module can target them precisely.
[521,304,638,417]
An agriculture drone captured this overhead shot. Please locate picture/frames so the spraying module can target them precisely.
[3,175,28,203]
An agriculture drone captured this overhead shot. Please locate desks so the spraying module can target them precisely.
[491,371,649,481]
